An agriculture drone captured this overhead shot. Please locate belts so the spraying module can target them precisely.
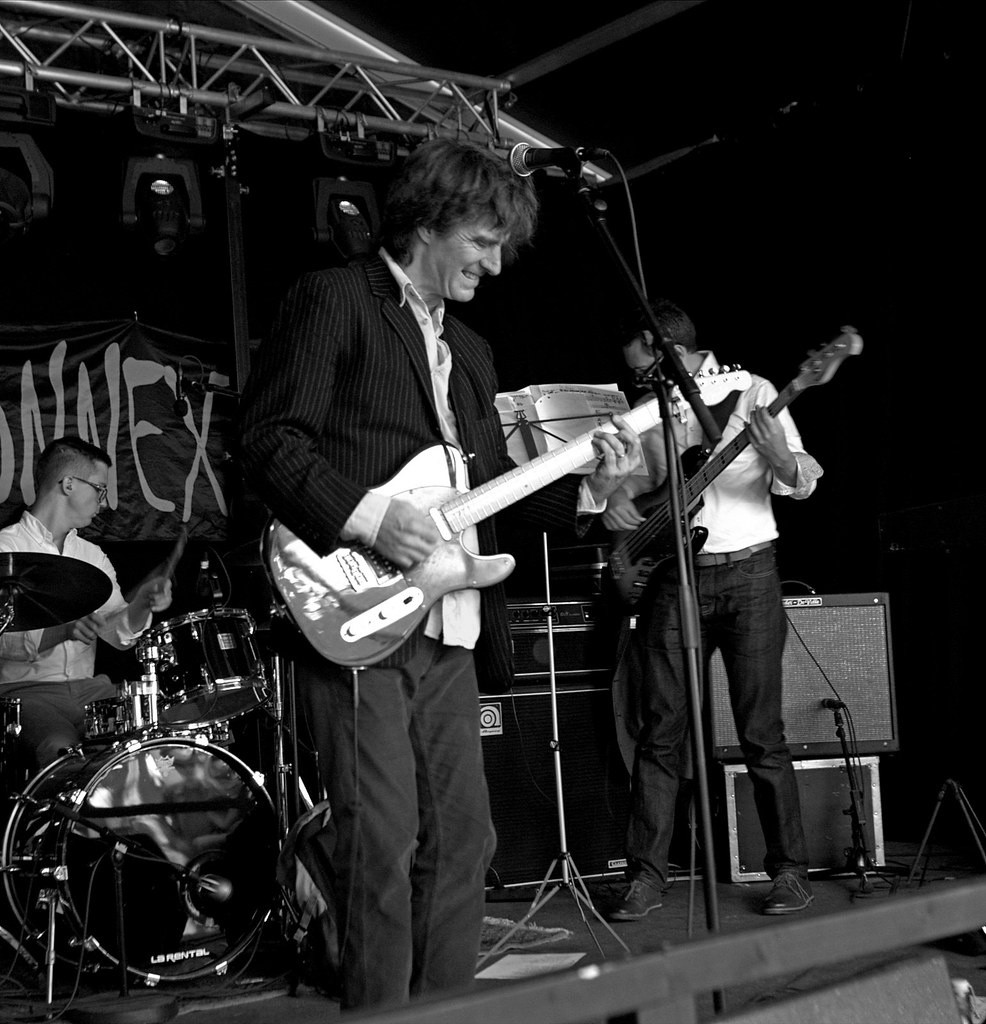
[695,541,773,567]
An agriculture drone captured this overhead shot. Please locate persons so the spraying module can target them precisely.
[0,438,174,792]
[602,302,823,922]
[227,144,645,1010]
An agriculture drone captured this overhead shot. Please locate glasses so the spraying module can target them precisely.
[58,475,108,503]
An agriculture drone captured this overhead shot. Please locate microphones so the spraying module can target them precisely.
[822,699,846,710]
[508,141,611,176]
[197,874,233,901]
[174,362,188,417]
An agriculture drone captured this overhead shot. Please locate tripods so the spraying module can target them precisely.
[806,709,921,895]
[475,531,634,973]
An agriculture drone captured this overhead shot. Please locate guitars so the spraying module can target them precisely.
[259,362,752,668]
[582,324,865,664]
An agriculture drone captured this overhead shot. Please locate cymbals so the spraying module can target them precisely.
[1,550,114,633]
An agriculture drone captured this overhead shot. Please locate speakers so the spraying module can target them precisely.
[706,592,900,761]
[475,685,640,890]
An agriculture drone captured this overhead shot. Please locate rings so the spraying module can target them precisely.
[617,455,628,460]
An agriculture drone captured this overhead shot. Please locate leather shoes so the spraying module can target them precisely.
[609,879,663,919]
[762,870,815,915]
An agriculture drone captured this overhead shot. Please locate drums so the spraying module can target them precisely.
[133,607,275,731]
[2,736,284,986]
[83,696,236,749]
